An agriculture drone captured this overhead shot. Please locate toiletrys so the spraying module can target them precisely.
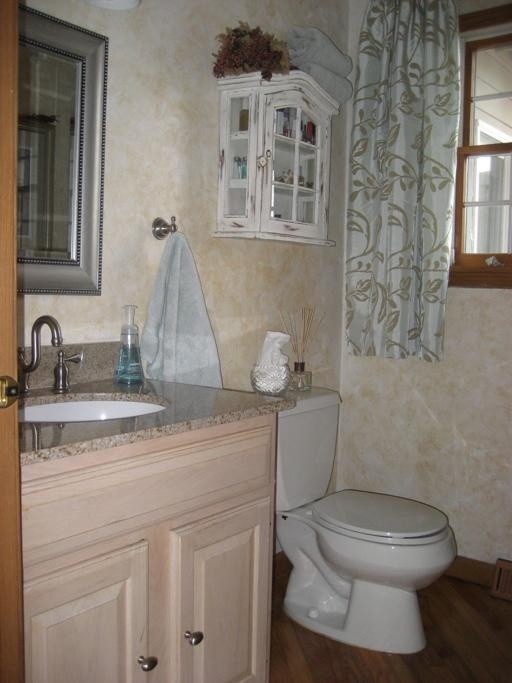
[232,156,247,177]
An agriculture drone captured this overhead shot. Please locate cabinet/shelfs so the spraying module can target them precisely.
[211,66,342,246]
[20,414,281,681]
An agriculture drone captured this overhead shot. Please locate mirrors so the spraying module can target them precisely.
[17,2,109,295]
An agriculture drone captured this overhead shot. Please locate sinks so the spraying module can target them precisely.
[19,393,172,423]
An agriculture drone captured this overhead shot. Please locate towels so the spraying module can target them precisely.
[138,230,223,391]
[281,23,356,108]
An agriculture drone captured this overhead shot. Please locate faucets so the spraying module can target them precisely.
[18,315,63,392]
[30,422,65,451]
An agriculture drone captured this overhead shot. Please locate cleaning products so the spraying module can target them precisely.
[114,305,145,385]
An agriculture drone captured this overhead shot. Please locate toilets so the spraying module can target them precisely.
[277,385,458,656]
